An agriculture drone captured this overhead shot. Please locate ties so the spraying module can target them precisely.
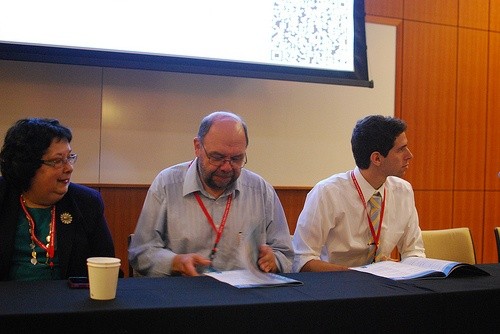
[368,194,382,263]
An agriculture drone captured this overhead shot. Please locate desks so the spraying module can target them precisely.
[0,264,500,334]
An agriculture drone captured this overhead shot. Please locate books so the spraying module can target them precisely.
[348,256,492,282]
[203,268,304,289]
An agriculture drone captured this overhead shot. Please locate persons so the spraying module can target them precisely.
[292,114,426,274]
[128,111,295,278]
[0,116,124,281]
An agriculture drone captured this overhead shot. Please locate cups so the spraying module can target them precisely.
[87,256,121,300]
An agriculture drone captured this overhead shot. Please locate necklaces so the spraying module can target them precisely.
[23,200,52,266]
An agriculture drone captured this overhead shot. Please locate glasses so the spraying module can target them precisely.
[40,154,77,168]
[200,140,247,169]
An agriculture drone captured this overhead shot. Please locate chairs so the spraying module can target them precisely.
[419,227,476,265]
